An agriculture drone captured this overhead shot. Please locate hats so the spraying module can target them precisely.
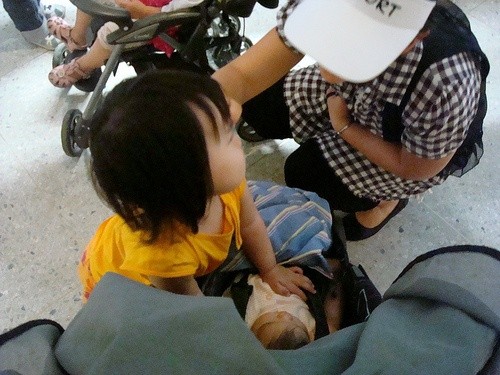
[283,0,437,83]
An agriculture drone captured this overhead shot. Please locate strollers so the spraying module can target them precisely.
[52,0,279,157]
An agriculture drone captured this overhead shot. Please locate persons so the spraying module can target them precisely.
[210,0,490,242]
[1,0,66,52]
[77,69,317,304]
[47,0,205,89]
[222,272,315,350]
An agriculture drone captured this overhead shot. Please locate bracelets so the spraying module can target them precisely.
[337,120,357,135]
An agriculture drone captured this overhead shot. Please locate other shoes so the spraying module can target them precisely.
[20,4,66,52]
[342,198,410,240]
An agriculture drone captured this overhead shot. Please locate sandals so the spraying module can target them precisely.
[49,57,91,88]
[48,17,89,51]
